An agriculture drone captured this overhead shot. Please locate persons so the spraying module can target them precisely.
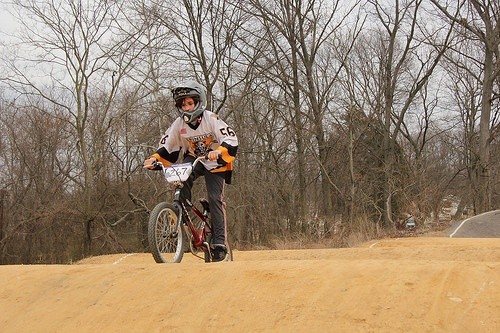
[143,81,239,262]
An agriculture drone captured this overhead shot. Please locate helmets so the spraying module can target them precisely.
[171,79,208,123]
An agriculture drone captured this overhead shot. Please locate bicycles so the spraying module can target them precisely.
[139,154,234,262]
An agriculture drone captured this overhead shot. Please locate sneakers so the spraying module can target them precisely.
[183,216,189,226]
[210,247,228,263]
